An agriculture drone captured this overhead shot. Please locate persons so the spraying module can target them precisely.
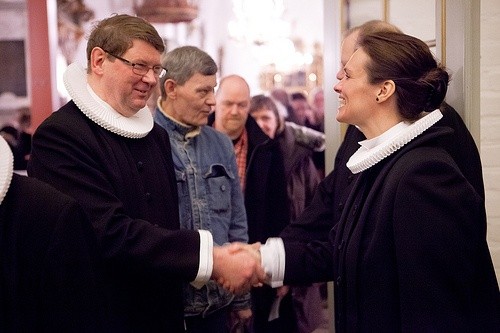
[29,12,262,333]
[0,113,85,333]
[153,47,252,333]
[206,20,500,333]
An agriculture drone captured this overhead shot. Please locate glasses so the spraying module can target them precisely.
[103,49,168,79]
